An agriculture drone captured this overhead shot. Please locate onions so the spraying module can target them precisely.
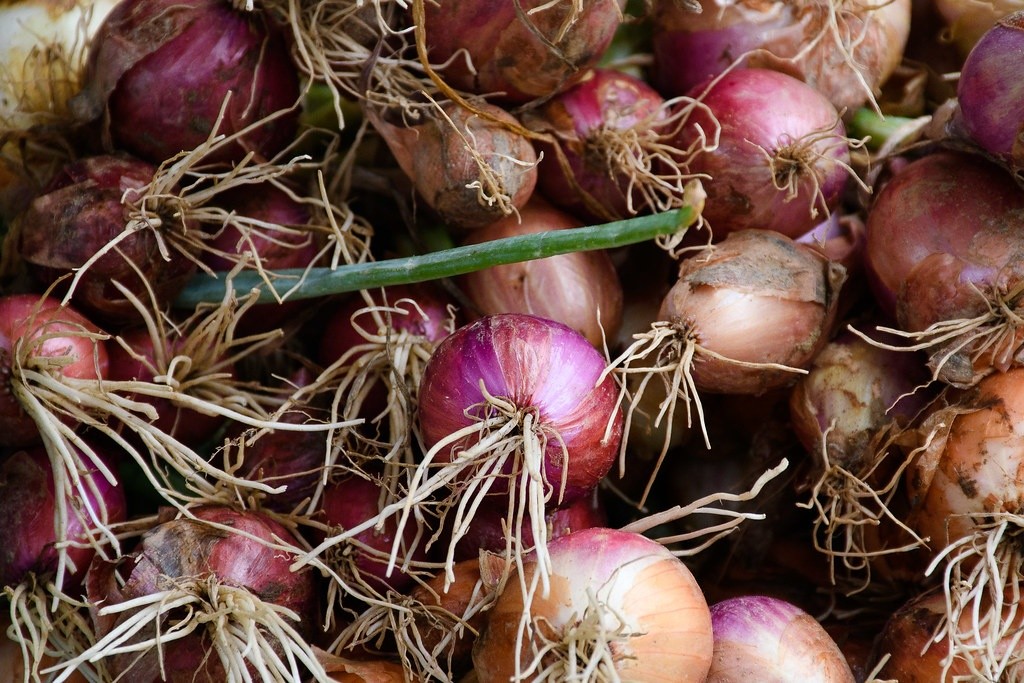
[0,0,1024,683]
